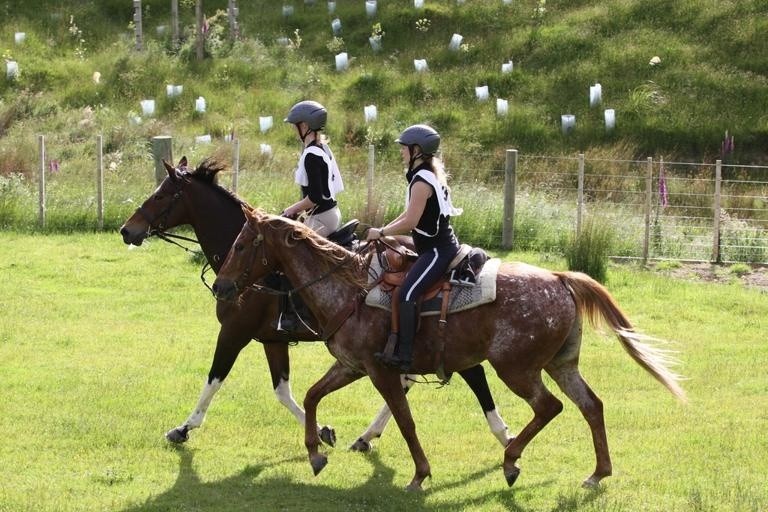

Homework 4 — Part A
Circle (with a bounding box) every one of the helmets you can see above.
[284,100,327,131]
[394,124,441,154]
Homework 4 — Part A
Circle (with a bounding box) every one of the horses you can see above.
[118,156,517,454]
[210,203,697,493]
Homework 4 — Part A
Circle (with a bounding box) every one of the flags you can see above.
[657,157,669,208]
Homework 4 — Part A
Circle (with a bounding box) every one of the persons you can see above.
[361,122,462,373]
[268,99,345,332]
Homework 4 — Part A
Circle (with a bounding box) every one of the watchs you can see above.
[378,227,386,239]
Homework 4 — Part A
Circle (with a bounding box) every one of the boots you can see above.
[373,301,420,373]
